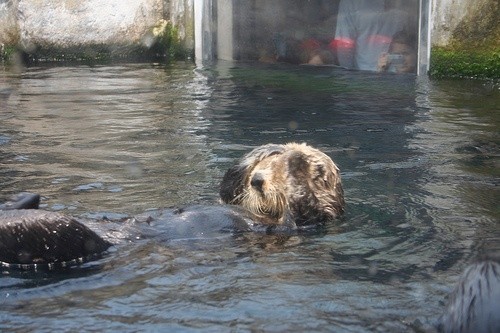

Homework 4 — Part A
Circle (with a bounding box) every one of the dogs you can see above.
[0,143,347,270]
